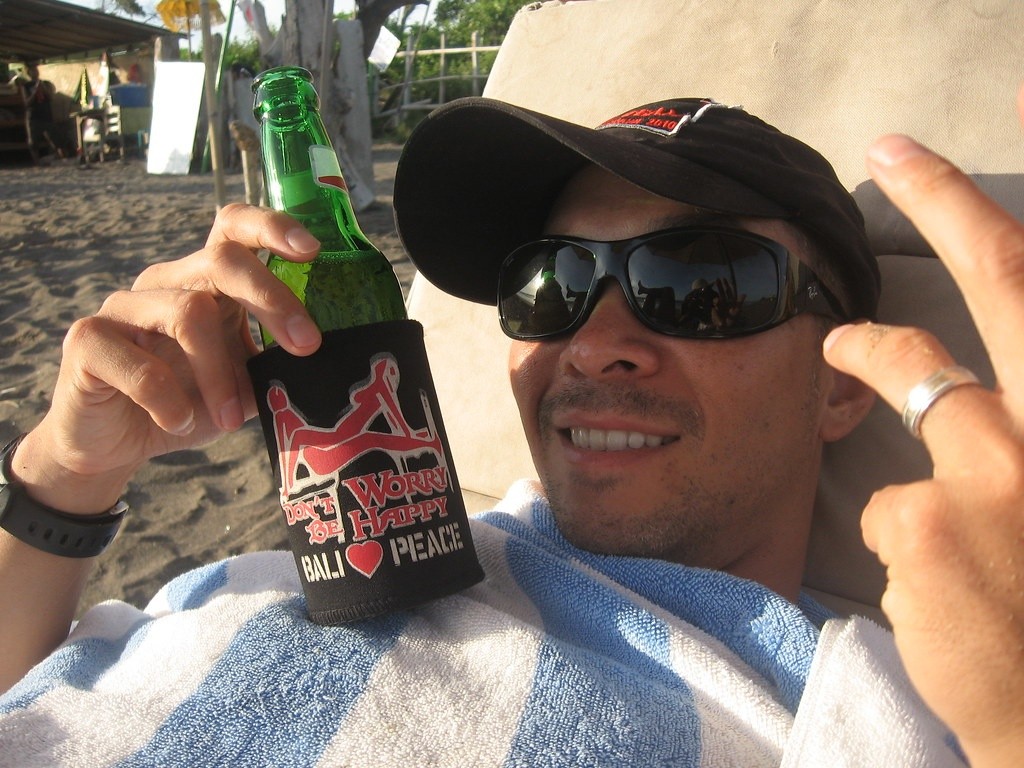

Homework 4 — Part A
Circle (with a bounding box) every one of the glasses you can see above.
[496,225,848,341]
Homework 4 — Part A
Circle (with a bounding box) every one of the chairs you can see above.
[0,85,55,167]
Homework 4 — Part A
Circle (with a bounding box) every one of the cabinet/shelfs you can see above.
[105,105,154,162]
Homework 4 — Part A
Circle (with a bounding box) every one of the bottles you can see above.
[243,65,485,626]
[536,249,563,303]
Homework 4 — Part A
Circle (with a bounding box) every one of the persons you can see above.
[0,98,1024,768]
[22,67,67,166]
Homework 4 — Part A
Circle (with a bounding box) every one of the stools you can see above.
[69,108,106,164]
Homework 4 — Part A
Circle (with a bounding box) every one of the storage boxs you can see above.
[108,81,149,106]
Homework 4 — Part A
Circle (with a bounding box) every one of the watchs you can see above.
[0,432,129,558]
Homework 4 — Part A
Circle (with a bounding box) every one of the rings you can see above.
[902,366,980,439]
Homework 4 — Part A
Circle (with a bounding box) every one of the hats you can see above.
[687,277,716,296]
[392,97,880,324]
